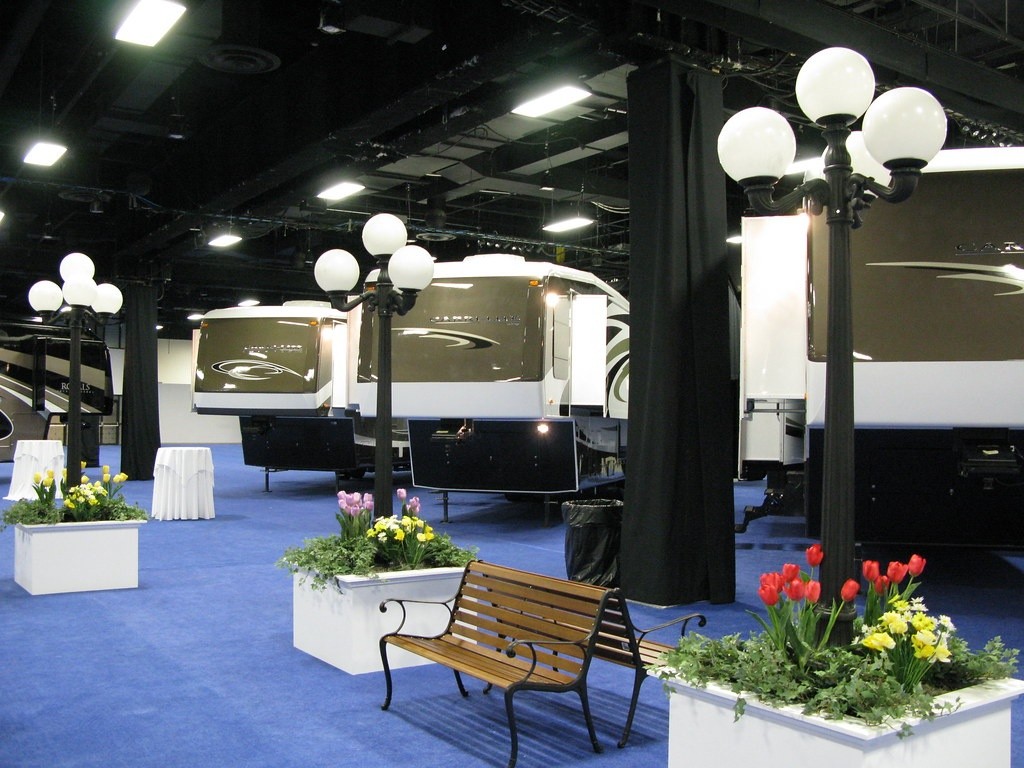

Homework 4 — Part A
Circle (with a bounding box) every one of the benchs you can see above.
[379,557,715,768]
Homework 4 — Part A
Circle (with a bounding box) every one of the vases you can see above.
[648,664,1024,767]
[286,561,478,675]
[12,519,146,596]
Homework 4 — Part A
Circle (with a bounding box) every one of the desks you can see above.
[153,447,216,521]
[7,439,66,500]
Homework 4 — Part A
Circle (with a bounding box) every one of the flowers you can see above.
[5,459,148,525]
[274,489,481,596]
[658,538,1021,743]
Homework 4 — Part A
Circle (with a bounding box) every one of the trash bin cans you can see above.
[81,413,101,468]
[561,497,623,591]
